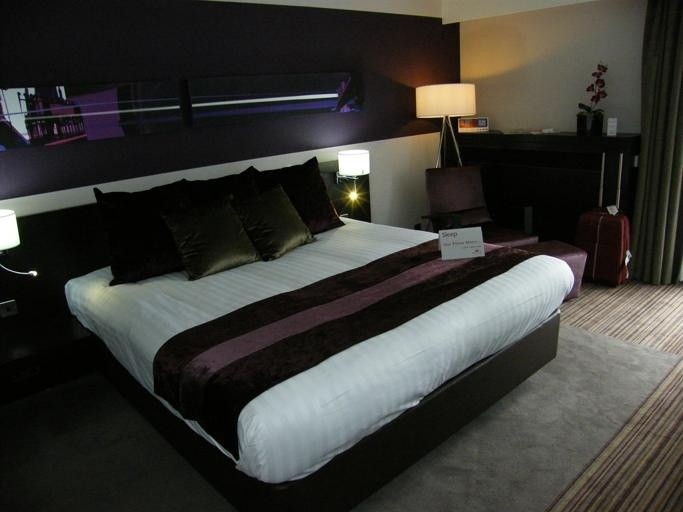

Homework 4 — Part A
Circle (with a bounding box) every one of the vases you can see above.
[577,115,604,135]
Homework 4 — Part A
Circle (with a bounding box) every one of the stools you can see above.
[486,227,588,303]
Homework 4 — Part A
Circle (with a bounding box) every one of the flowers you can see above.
[577,59,608,118]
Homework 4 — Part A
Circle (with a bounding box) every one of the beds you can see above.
[64,214,577,511]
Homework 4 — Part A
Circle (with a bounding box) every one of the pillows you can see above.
[93,157,345,286]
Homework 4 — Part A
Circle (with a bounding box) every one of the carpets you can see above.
[1,321,682,512]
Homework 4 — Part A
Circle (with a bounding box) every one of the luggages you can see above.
[578,149,636,289]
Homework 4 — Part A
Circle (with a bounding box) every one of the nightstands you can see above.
[0,271,68,406]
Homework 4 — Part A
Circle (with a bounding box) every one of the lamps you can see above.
[415,82,476,167]
[335,149,370,184]
[0,209,39,278]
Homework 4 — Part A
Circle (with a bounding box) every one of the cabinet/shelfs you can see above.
[440,131,642,247]
